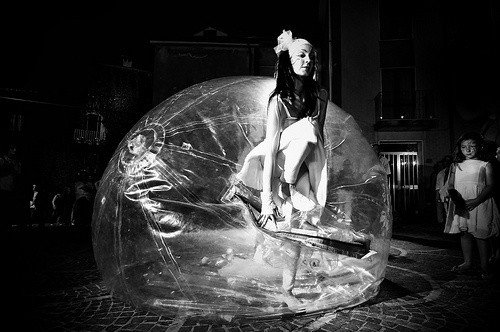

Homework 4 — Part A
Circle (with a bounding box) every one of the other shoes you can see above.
[276,184,317,211]
[280,293,306,313]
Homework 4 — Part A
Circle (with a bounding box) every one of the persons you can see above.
[28,176,101,235]
[433,123,500,279]
[235,29,330,314]
